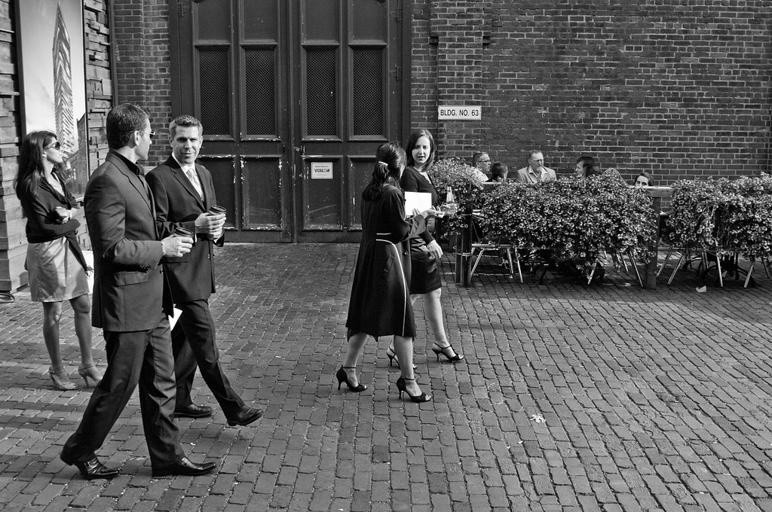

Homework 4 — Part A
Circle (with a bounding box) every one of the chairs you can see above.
[587,249,643,288]
[461,214,523,283]
[657,212,723,287]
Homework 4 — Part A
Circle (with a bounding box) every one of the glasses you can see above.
[140,130,156,138]
[44,142,60,150]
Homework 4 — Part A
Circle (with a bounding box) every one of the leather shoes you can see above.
[152,456,216,476]
[228,405,263,427]
[174,404,212,417]
[61,453,119,479]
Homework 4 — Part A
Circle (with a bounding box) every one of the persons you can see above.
[383,128,464,368]
[335,140,435,403]
[57,100,218,479]
[462,149,656,269]
[144,112,265,428]
[12,130,106,392]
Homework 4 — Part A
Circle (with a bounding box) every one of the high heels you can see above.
[396,377,431,402]
[432,342,464,362]
[387,346,417,369]
[48,368,78,390]
[336,365,367,392]
[78,366,103,388]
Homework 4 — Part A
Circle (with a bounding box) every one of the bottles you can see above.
[446,186,457,215]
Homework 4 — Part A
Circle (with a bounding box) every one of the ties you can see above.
[186,168,205,201]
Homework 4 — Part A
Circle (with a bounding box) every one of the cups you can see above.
[173,226,194,236]
[209,204,226,216]
